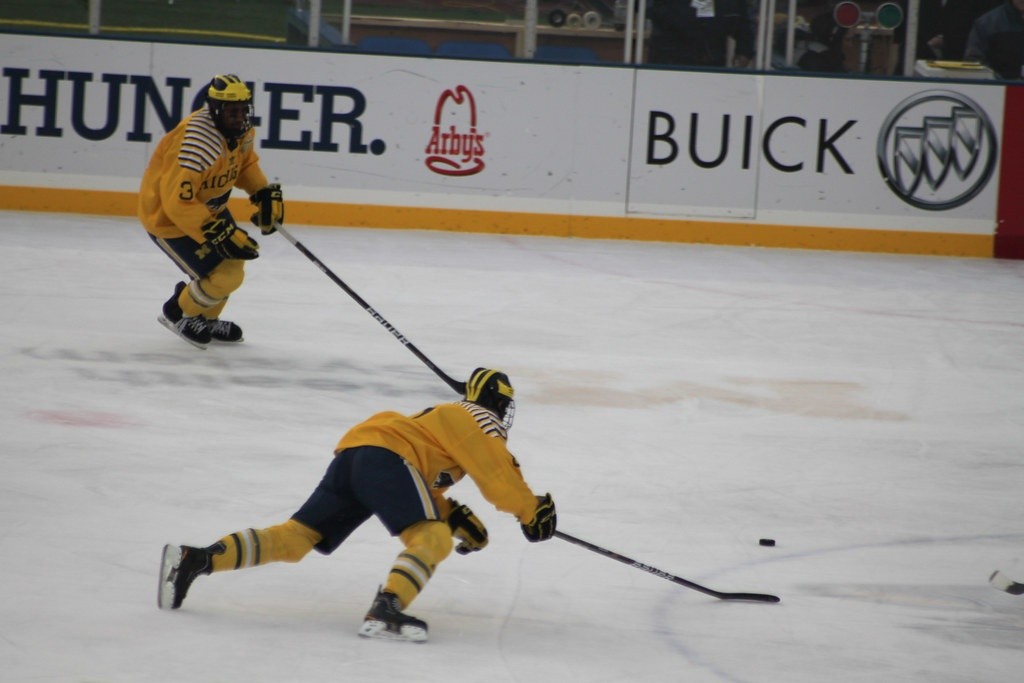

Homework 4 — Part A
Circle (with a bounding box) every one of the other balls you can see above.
[758,539,775,546]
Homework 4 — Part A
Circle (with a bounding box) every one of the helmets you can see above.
[206,73,252,137]
[465,367,516,430]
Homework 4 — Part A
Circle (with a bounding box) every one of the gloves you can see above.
[249,183,285,235]
[200,207,260,259]
[520,492,557,543]
[446,497,489,555]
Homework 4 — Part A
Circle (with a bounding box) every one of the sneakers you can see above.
[156,281,212,351]
[357,584,428,643]
[157,543,213,610]
[207,320,243,342]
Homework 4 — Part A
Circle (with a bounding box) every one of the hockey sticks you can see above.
[274,219,467,396]
[552,524,783,603]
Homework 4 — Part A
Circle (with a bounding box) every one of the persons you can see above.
[890,0,1024,79]
[135,73,285,352]
[633,0,755,69]
[156,367,557,645]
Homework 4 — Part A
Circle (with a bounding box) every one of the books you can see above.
[914,59,994,79]
